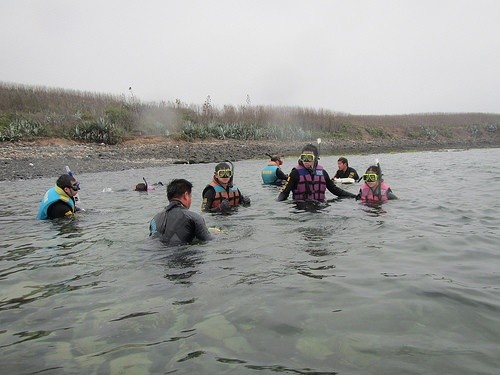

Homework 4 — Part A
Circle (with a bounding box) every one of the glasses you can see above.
[67,182,80,191]
[278,159,283,165]
[299,154,315,161]
[362,174,380,182]
[215,169,232,178]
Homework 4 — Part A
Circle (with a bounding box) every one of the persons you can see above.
[149,179,211,246]
[356,165,398,200]
[261,155,287,185]
[38,175,81,221]
[330,157,359,181]
[276,144,356,201]
[201,162,251,213]
